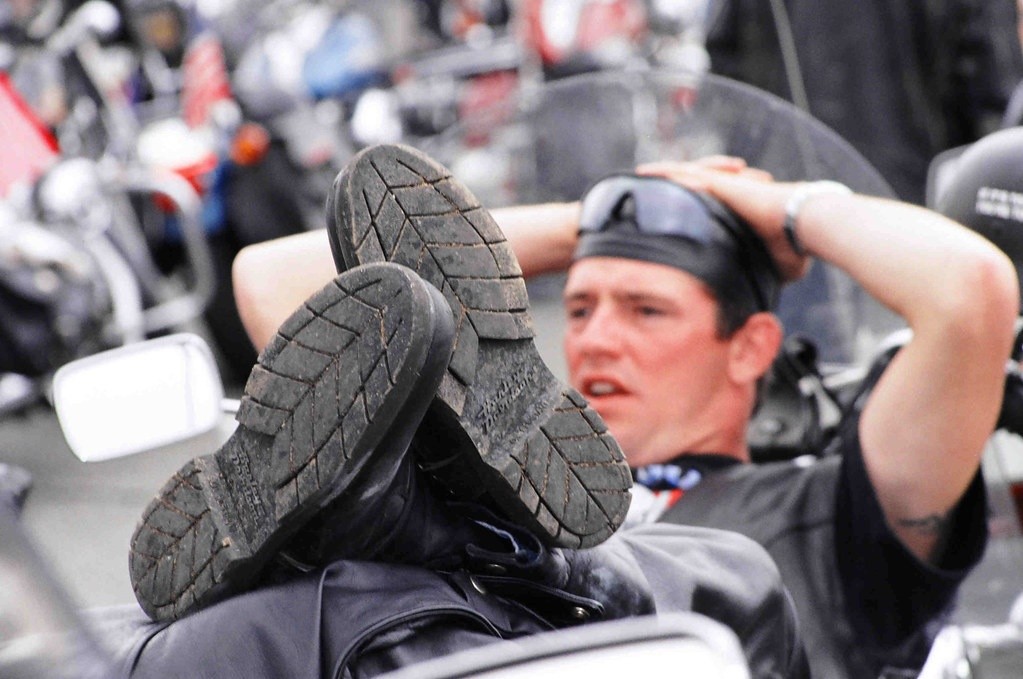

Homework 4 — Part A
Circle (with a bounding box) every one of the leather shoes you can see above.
[326,144,634,550]
[128,261,456,622]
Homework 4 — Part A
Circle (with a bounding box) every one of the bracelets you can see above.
[783,179,852,257]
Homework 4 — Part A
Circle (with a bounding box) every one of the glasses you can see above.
[575,174,737,252]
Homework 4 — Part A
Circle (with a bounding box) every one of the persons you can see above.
[127,142,1018,679]
